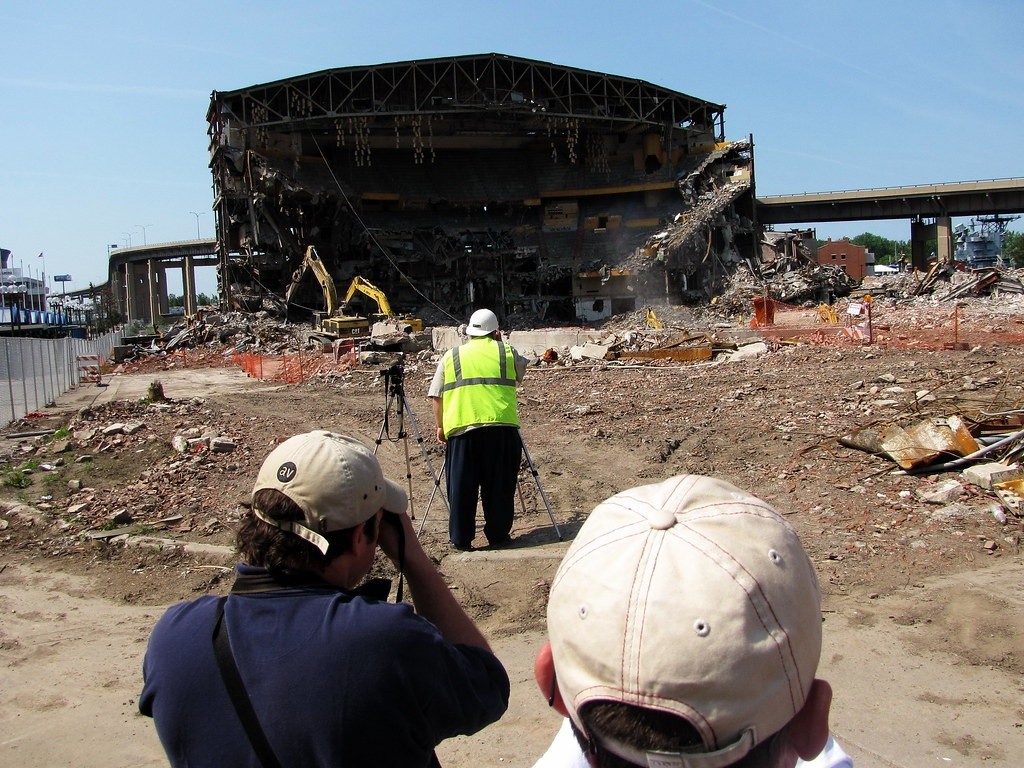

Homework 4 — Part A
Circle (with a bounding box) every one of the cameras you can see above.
[379,364,404,377]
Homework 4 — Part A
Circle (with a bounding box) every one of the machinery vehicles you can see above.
[283,245,373,354]
[345,276,424,333]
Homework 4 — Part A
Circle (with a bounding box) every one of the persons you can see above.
[524,472,855,767]
[136,429,511,768]
[427,307,530,551]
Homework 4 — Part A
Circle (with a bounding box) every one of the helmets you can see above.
[466,308,498,337]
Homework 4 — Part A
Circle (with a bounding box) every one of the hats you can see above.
[251,429,410,556]
[546,473,822,768]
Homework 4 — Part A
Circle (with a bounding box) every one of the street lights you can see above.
[47,297,63,339]
[73,299,86,338]
[85,305,95,340]
[189,210,207,239]
[62,295,77,338]
[135,223,153,247]
[0,274,27,338]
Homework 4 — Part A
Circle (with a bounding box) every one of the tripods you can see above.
[373,377,563,543]
